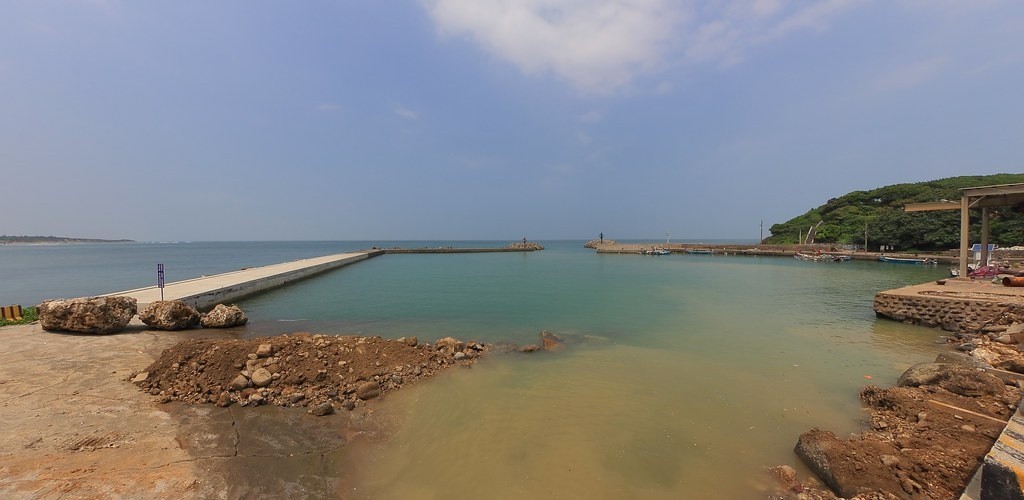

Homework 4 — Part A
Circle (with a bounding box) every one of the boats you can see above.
[638,248,670,255]
[950,263,978,278]
[794,252,851,261]
[876,253,937,264]
[685,248,715,254]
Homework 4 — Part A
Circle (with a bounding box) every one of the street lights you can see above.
[599,232,604,244]
